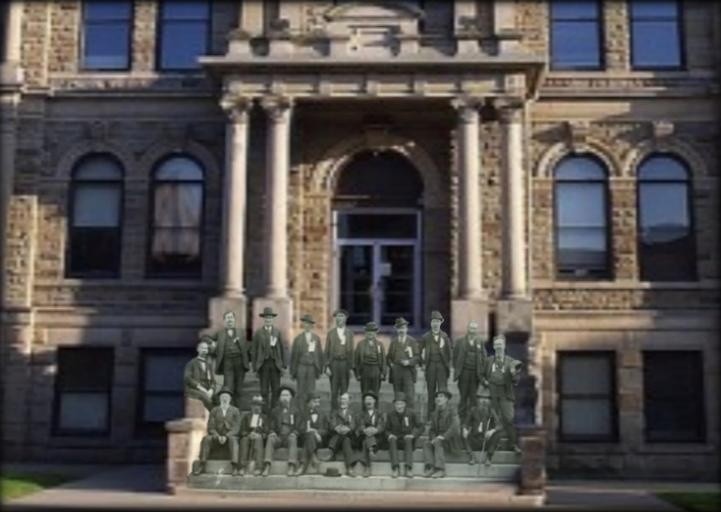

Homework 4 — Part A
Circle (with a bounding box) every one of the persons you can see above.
[184,307,522,479]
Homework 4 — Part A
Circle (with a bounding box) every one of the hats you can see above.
[301,314,314,322]
[259,308,277,318]
[430,311,444,322]
[395,318,407,328]
[217,386,233,396]
[363,322,378,332]
[277,383,295,398]
[393,392,407,401]
[364,391,378,399]
[325,467,340,477]
[250,394,265,404]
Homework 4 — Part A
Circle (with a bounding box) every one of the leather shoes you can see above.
[233,463,270,477]
[392,466,445,478]
[287,463,305,475]
[193,463,204,476]
